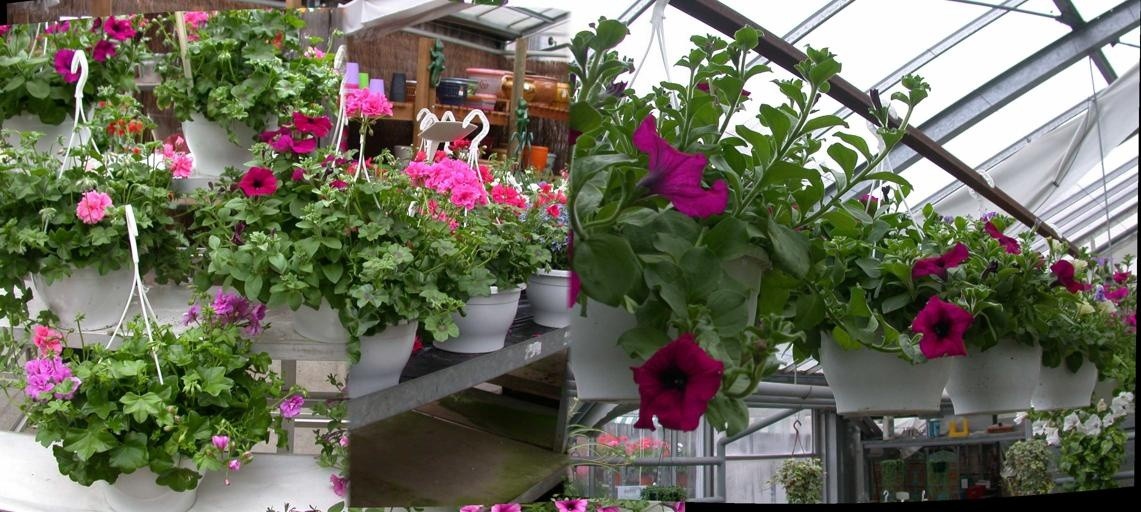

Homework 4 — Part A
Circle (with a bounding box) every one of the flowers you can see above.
[568,17,1137,497]
[0,5,568,497]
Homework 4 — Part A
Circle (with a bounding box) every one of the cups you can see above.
[343,59,416,101]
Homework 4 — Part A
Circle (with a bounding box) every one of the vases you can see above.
[101,456,206,512]
